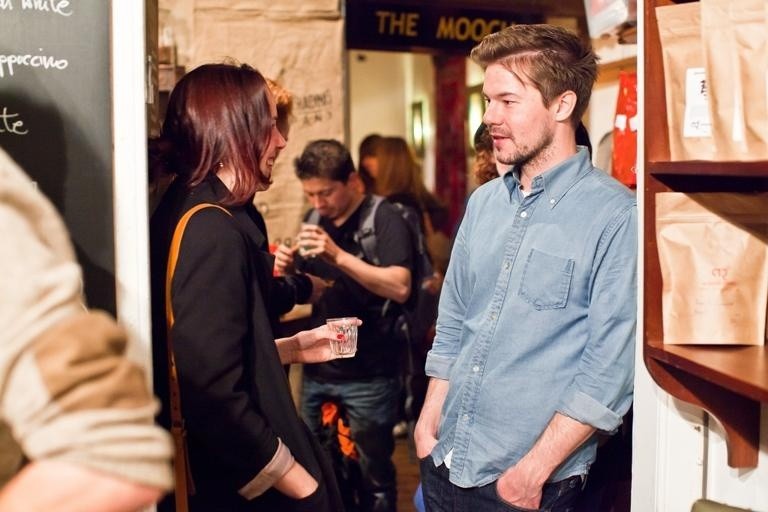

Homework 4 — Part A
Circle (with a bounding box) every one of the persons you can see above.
[407,22,634,510]
[574,119,593,159]
[0,143,177,511]
[150,57,364,511]
[271,273,328,321]
[372,131,445,436]
[273,136,417,512]
[471,125,499,184]
[358,134,384,193]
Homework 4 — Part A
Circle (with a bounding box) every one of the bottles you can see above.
[612,70,640,190]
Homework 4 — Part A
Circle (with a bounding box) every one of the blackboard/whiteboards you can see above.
[0,0,116,319]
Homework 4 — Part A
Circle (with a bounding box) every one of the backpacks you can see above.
[306,192,440,347]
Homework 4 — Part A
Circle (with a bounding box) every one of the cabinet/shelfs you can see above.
[640,1,768,468]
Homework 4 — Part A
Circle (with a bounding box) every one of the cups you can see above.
[325,317,359,356]
